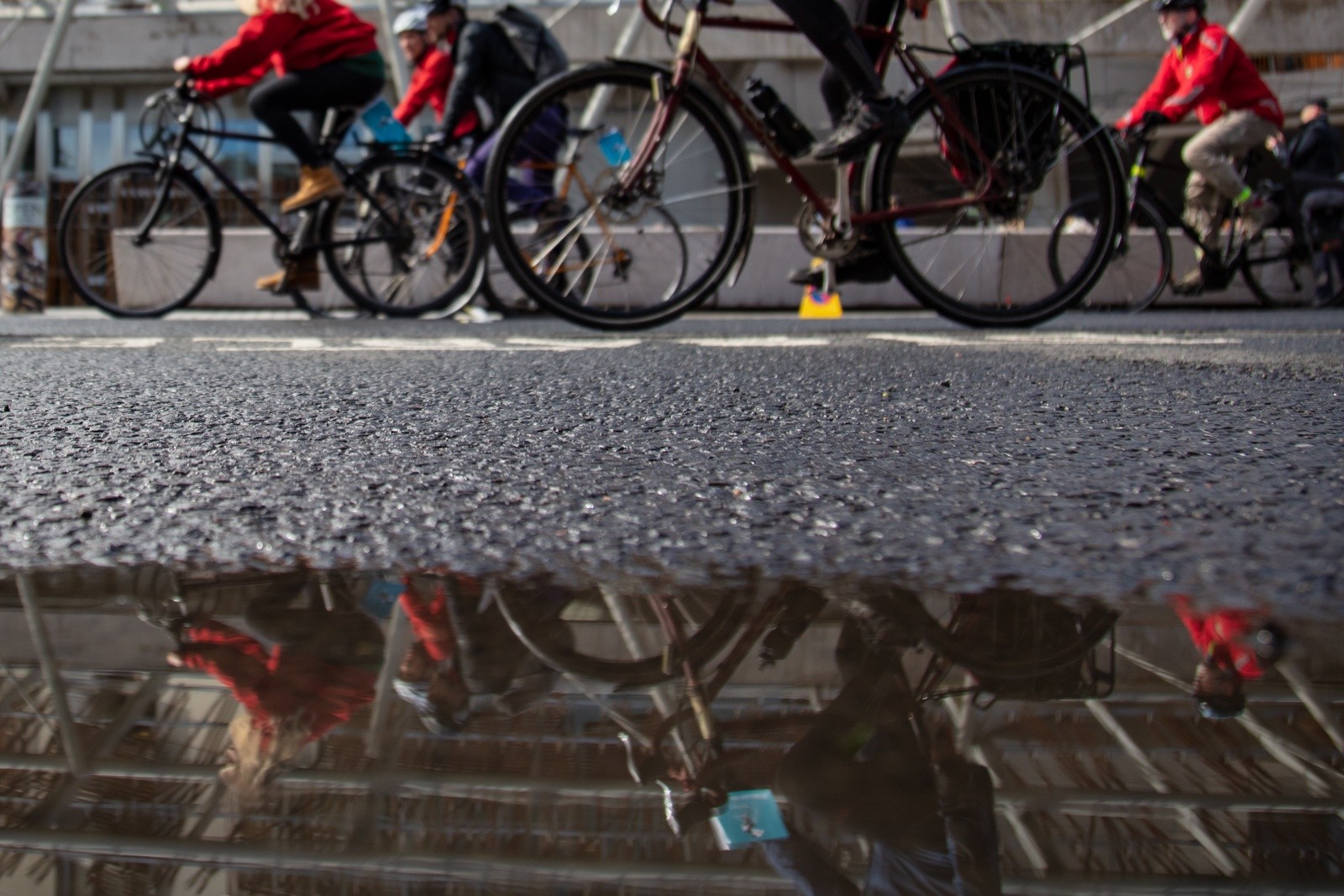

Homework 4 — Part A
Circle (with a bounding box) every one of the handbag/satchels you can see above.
[935,38,1063,194]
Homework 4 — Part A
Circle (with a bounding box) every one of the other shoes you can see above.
[1177,266,1219,292]
[1246,198,1275,240]
[280,165,343,214]
[256,262,320,291]
[533,202,572,243]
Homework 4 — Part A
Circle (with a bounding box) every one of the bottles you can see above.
[744,74,818,156]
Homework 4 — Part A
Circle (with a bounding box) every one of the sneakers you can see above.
[788,246,894,285]
[815,95,894,156]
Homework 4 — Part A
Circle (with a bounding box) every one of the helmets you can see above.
[417,692,479,734]
[392,7,428,37]
[1192,690,1246,719]
[393,677,432,706]
[423,0,468,16]
[1151,1,1207,14]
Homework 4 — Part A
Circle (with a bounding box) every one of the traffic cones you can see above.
[796,251,847,322]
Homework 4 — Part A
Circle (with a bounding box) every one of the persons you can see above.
[1270,100,1331,168]
[174,1,388,289]
[1115,0,1284,286]
[675,600,1002,895]
[392,1,571,242]
[770,0,895,283]
[1173,595,1302,716]
[393,571,577,734]
[166,575,387,792]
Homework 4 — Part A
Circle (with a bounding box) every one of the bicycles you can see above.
[52,67,486,324]
[87,572,1122,839]
[483,0,1133,337]
[279,0,692,327]
[1049,120,1344,313]
